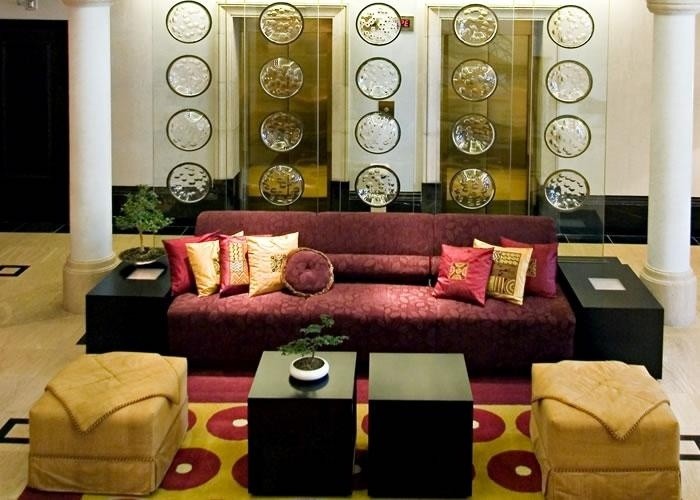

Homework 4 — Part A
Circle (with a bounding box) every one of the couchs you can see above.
[87,209,663,380]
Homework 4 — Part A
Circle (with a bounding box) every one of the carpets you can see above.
[15,367,544,499]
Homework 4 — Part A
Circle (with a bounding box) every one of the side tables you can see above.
[85,256,171,355]
[557,257,664,379]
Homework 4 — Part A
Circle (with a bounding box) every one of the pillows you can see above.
[185,230,244,297]
[280,247,334,297]
[500,236,557,298]
[473,238,534,306]
[216,234,274,296]
[432,244,494,307]
[162,230,220,297]
[246,231,299,297]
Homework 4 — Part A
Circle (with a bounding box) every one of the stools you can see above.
[530,360,682,500]
[28,351,187,496]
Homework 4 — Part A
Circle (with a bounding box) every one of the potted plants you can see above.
[278,313,349,381]
[113,184,175,267]
